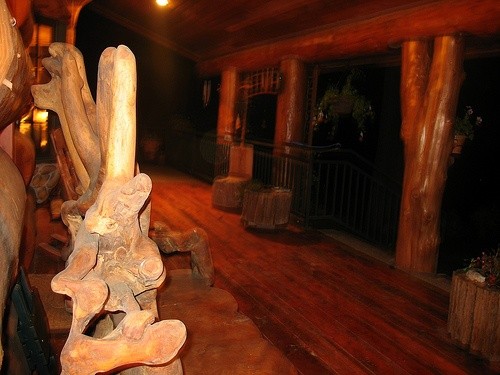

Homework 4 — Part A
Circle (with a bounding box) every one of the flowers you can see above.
[455,104,482,135]
[467,247,500,286]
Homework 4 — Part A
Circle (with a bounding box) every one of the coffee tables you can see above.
[448,267,499,362]
[213,176,245,214]
[243,185,292,231]
[139,135,160,162]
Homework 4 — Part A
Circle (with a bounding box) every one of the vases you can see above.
[451,135,467,154]
